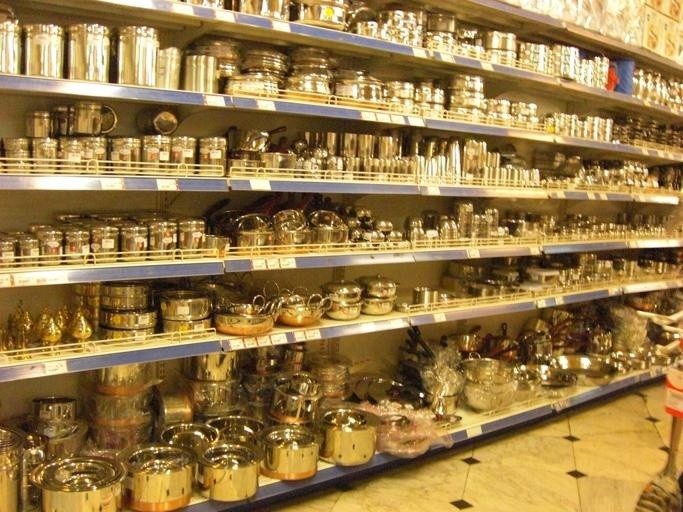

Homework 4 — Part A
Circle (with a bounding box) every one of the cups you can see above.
[1,1,683,268]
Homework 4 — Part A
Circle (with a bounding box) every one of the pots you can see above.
[1,249,683,512]
